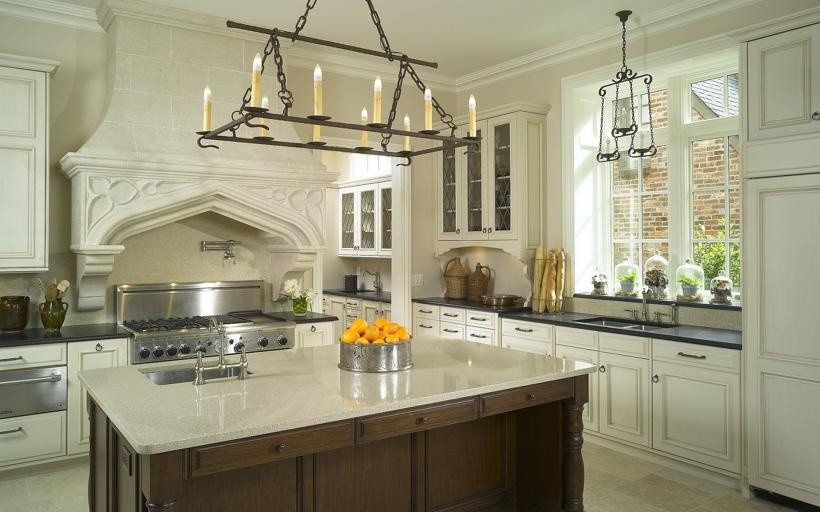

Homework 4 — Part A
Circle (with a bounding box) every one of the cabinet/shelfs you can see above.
[432,111,548,306]
[84,375,587,512]
[741,21,820,139]
[741,141,820,512]
[320,294,391,336]
[0,54,62,273]
[335,180,392,258]
[414,304,498,349]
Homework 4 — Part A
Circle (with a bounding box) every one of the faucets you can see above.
[208,316,226,364]
[641,288,653,321]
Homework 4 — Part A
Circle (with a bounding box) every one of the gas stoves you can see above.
[117,310,296,364]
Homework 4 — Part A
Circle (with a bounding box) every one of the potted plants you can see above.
[279,279,313,316]
[591,257,733,306]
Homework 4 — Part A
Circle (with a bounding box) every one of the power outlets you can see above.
[413,273,424,287]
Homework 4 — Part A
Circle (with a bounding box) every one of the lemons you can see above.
[341,317,410,344]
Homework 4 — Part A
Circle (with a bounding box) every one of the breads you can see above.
[532,245,565,313]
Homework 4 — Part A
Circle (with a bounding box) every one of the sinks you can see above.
[574,317,678,332]
[137,360,254,386]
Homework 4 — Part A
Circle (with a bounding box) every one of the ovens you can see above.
[0,365,68,423]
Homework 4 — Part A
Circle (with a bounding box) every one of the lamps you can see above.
[595,9,657,161]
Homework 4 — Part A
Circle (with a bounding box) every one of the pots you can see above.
[480,294,522,307]
